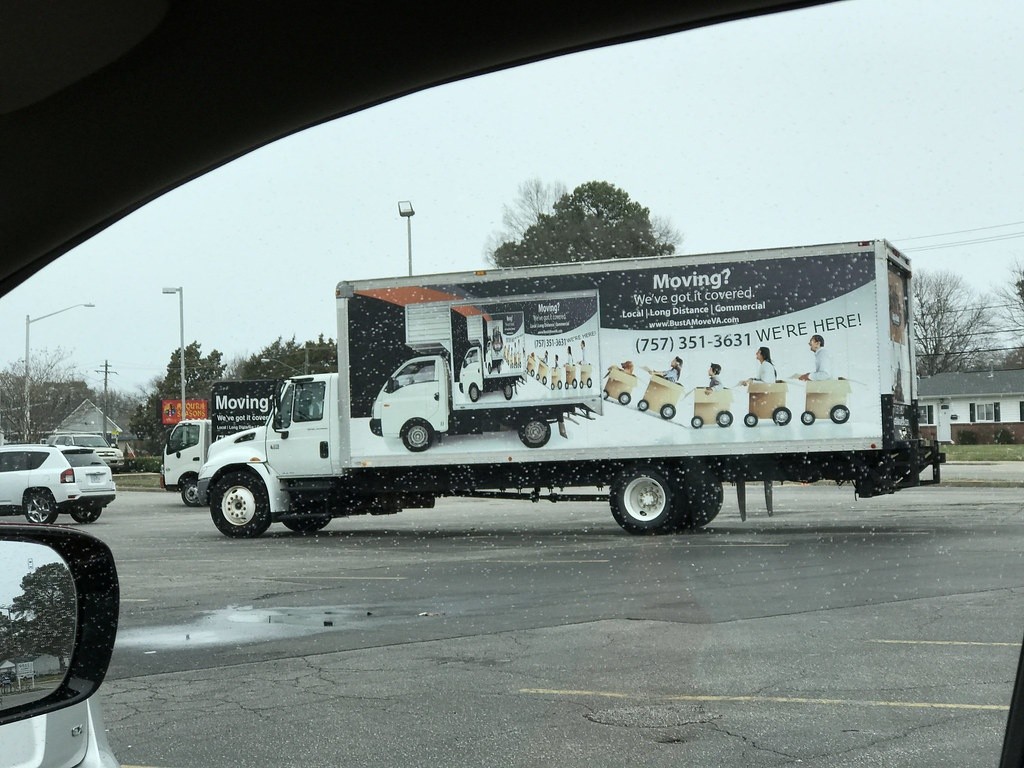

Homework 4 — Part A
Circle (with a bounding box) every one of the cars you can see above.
[46,434,125,474]
[0,444,118,524]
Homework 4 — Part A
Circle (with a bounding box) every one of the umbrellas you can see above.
[1,661,15,670]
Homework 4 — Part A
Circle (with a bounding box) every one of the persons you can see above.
[744,347,777,386]
[705,363,723,395]
[798,334,837,382]
[540,340,588,368]
[508,339,520,355]
[649,356,683,384]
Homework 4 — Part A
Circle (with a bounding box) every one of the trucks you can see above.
[159,378,283,507]
[194,236,918,537]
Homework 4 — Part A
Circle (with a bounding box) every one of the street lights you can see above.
[25,303,95,443]
[162,286,185,421]
[398,200,416,276]
[260,357,308,376]
